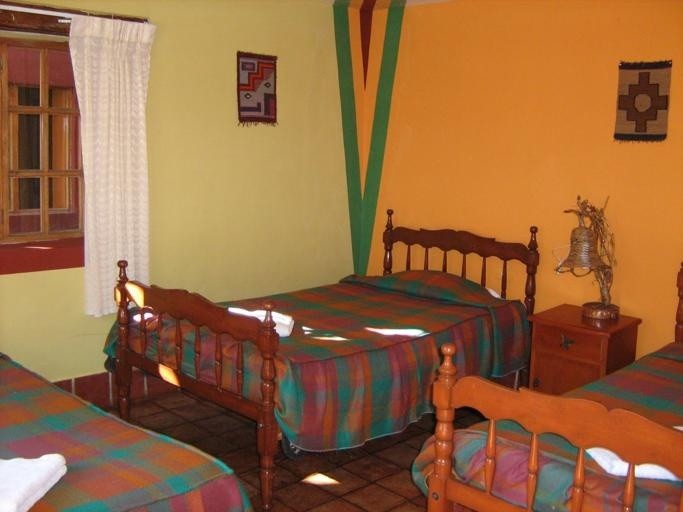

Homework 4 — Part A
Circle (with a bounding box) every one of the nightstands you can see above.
[527,303,643,397]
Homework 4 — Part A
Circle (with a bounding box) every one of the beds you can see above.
[0,353,253,512]
[411,262,683,512]
[114,208,540,512]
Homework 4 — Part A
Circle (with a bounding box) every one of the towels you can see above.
[0,453,67,511]
[586,426,683,482]
[227,306,295,337]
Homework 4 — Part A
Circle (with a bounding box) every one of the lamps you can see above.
[552,194,621,320]
